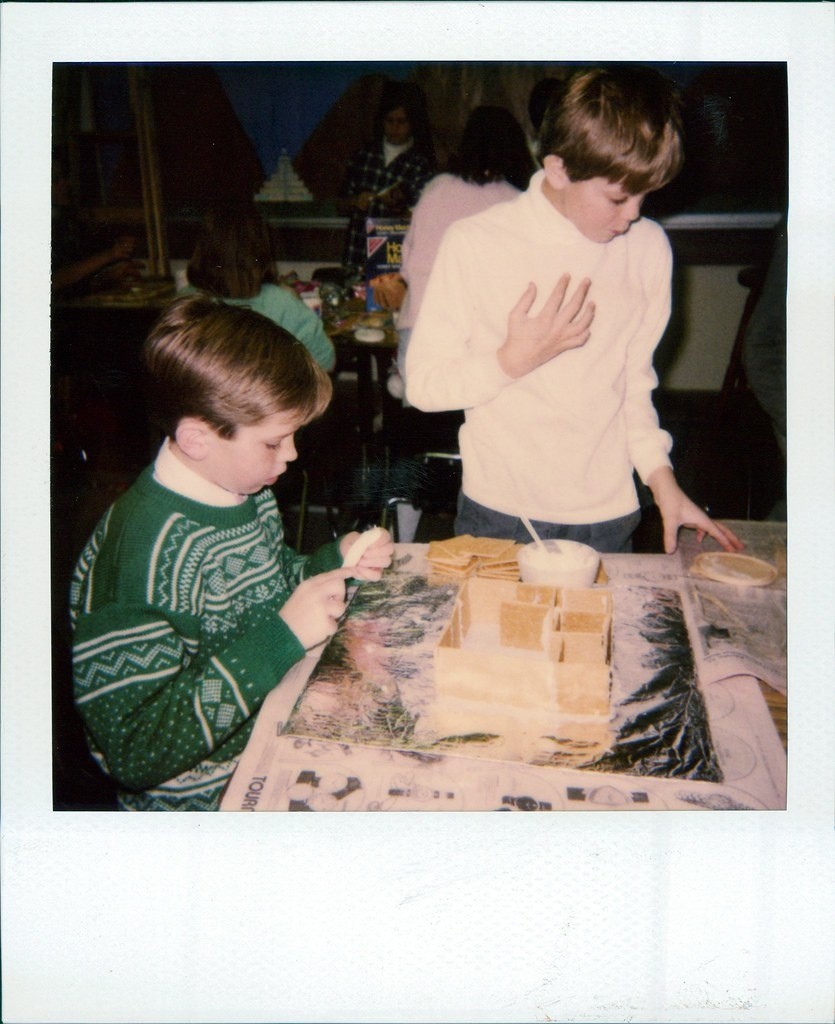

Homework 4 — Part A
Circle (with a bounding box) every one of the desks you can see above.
[217,519,787,811]
[52,277,399,351]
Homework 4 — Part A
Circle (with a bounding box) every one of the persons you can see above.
[344,86,437,282]
[527,77,565,156]
[728,220,786,480]
[354,107,535,544]
[71,297,397,812]
[52,148,165,443]
[157,198,337,503]
[405,70,742,556]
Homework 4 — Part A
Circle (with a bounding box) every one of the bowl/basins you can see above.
[518,539,600,588]
